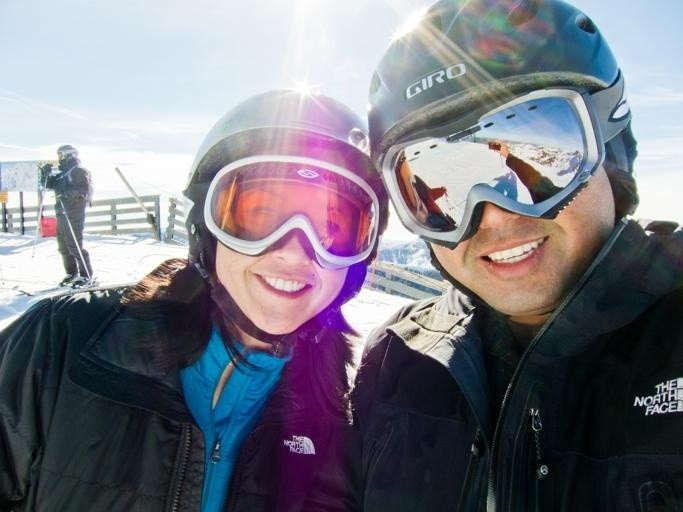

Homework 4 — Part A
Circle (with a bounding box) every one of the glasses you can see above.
[199,154,381,271]
[383,86,606,247]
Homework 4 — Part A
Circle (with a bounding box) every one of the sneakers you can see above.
[63,271,92,288]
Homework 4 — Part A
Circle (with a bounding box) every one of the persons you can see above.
[350,1,683,511]
[0,85,390,511]
[486,138,565,205]
[39,144,93,288]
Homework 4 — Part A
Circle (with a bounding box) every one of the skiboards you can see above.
[18,277,102,299]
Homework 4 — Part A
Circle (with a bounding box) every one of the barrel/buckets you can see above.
[39,216,58,238]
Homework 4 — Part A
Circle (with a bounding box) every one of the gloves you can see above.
[40,164,54,175]
[60,191,77,205]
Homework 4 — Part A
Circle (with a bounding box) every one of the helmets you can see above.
[369,0,631,164]
[184,89,389,230]
[57,146,78,161]
